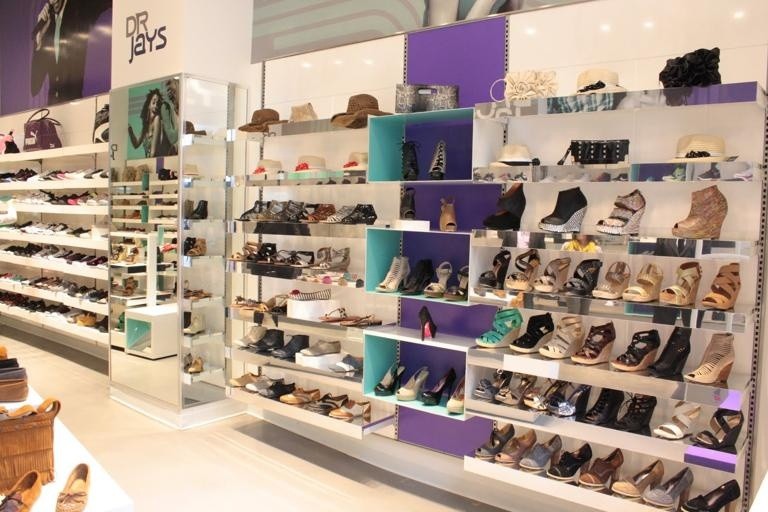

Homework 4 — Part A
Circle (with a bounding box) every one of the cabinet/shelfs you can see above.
[366,106,471,440]
[0,142,111,409]
[111,177,180,361]
[225,120,403,442]
[465,80,765,510]
[185,134,224,385]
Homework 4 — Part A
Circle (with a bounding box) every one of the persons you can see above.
[161,78,179,155]
[30,0,112,106]
[127,89,167,157]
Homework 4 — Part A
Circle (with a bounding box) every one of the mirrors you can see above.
[111,74,181,407]
[183,77,249,404]
[125,316,153,355]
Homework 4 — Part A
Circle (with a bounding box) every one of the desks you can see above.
[0,379,156,512]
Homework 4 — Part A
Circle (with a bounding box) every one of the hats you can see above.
[663,134,739,164]
[293,155,336,174]
[330,94,394,129]
[489,143,542,167]
[572,69,626,95]
[185,121,207,135]
[251,159,283,175]
[290,102,320,122]
[238,109,288,132]
[342,152,368,171]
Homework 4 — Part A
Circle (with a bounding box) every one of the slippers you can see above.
[318,308,360,322]
[190,289,212,300]
[184,288,193,297]
[340,314,382,326]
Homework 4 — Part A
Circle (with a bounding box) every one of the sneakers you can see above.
[11,221,91,237]
[16,190,109,206]
[590,172,611,182]
[539,175,557,184]
[479,173,494,182]
[611,174,629,181]
[507,172,527,182]
[662,168,685,181]
[574,173,589,182]
[558,173,574,183]
[734,170,753,181]
[697,167,721,180]
[492,173,511,181]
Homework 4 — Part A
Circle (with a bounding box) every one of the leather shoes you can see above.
[245,376,284,392]
[309,393,349,410]
[280,387,320,404]
[101,171,110,179]
[271,335,309,359]
[184,353,192,368]
[65,169,88,179]
[300,340,341,356]
[66,253,89,261]
[237,327,267,348]
[258,381,295,399]
[31,244,58,259]
[0,269,69,292]
[328,354,363,373]
[188,356,203,373]
[91,171,106,179]
[57,173,64,178]
[87,256,107,265]
[84,169,103,179]
[67,311,108,334]
[0,358,19,367]
[48,248,74,260]
[329,400,370,419]
[0,367,26,378]
[70,273,108,303]
[44,170,62,179]
[0,470,42,512]
[184,312,191,328]
[2,243,42,257]
[0,172,15,182]
[27,173,45,181]
[0,379,28,402]
[248,329,284,351]
[18,169,36,181]
[56,464,89,512]
[0,292,70,315]
[229,372,262,387]
[184,316,205,334]
[12,168,29,181]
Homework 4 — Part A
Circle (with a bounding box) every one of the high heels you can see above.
[483,183,526,231]
[610,329,661,372]
[397,142,419,180]
[429,141,447,180]
[375,256,411,293]
[611,460,664,498]
[578,448,624,486]
[475,423,515,460]
[681,479,740,512]
[443,265,469,301]
[524,379,575,411]
[374,360,406,396]
[684,333,735,384]
[255,295,287,312]
[614,392,657,432]
[111,245,128,263]
[595,189,646,237]
[659,262,701,306]
[571,321,615,364]
[538,186,587,233]
[519,435,562,470]
[420,306,437,340]
[548,385,592,416]
[547,443,592,480]
[439,196,457,232]
[562,259,603,295]
[495,429,537,462]
[423,261,453,298]
[643,466,694,507]
[509,312,554,354]
[585,388,625,424]
[538,316,584,359]
[504,249,540,290]
[690,409,744,449]
[532,257,572,292]
[654,401,702,440]
[476,308,522,348]
[646,327,692,378]
[592,261,631,299]
[672,185,727,240]
[126,247,139,262]
[473,369,513,399]
[400,259,433,294]
[400,187,416,219]
[700,264,740,310]
[622,262,662,302]
[422,368,456,405]
[396,366,430,401]
[494,373,537,405]
[447,376,464,413]
[479,250,511,290]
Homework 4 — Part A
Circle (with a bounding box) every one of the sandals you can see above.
[242,299,256,310]
[257,250,296,264]
[319,206,355,224]
[235,200,270,221]
[228,241,261,261]
[288,204,319,223]
[184,237,196,255]
[269,200,303,222]
[244,243,276,262]
[185,201,207,219]
[274,251,314,266]
[290,248,332,268]
[185,200,194,217]
[229,296,245,307]
[341,204,377,225]
[299,204,335,223]
[186,239,207,256]
[310,247,350,271]
[270,306,286,314]
[288,288,331,301]
[249,200,288,222]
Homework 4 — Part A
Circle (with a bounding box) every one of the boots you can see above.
[123,278,134,295]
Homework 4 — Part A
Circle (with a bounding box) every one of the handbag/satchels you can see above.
[490,71,558,103]
[92,104,110,144]
[0,398,61,494]
[23,109,62,152]
[557,140,633,165]
[659,47,720,88]
[395,83,459,113]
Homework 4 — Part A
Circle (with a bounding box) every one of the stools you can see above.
[123,303,179,362]
